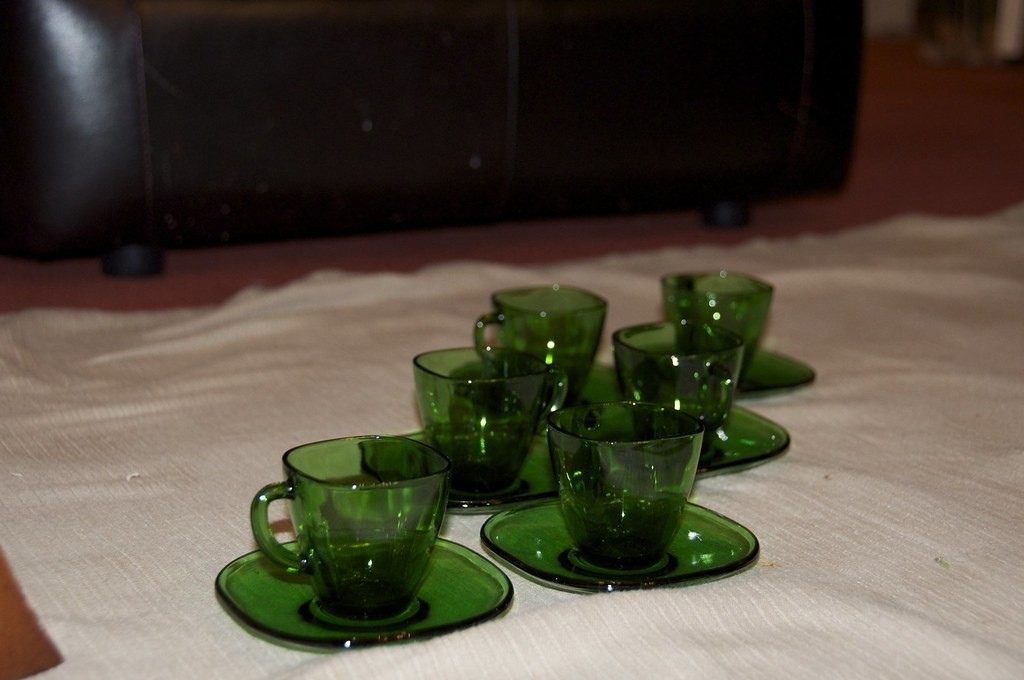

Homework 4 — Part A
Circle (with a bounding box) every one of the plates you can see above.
[581,341,817,482]
[478,494,761,596]
[397,432,560,517]
[215,536,516,650]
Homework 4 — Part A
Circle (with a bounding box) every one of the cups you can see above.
[411,345,567,498]
[612,320,747,463]
[473,285,609,398]
[661,268,775,382]
[250,433,454,620]
[546,401,706,571]
